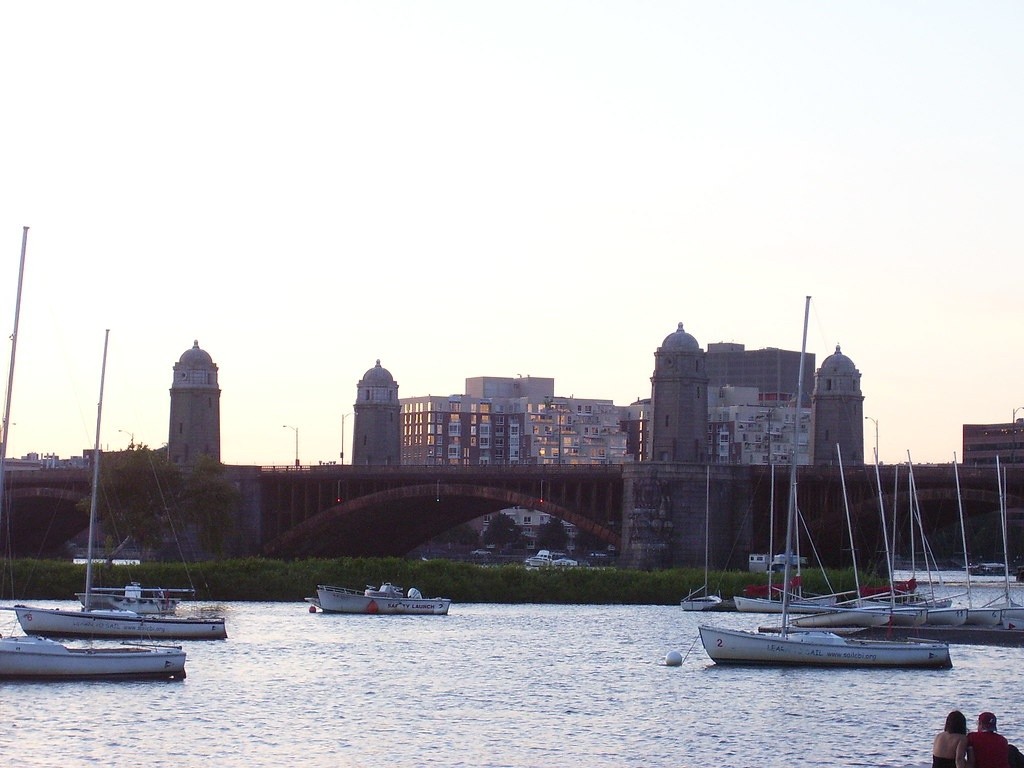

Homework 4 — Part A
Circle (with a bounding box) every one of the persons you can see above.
[932,710,1024,768]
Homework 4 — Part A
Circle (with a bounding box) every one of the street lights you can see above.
[1012,406,1024,470]
[282,425,300,469]
[864,416,879,464]
[339,412,360,465]
[118,429,134,451]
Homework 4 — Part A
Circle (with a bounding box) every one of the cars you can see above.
[472,549,492,553]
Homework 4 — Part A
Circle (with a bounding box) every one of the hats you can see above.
[978,712,998,731]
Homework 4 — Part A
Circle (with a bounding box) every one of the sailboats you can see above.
[696,295,954,669]
[732,443,1024,629]
[12,327,228,640]
[0,225,188,685]
[680,466,724,612]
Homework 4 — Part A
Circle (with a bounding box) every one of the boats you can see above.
[303,582,451,615]
[523,550,578,567]
[72,581,197,615]
